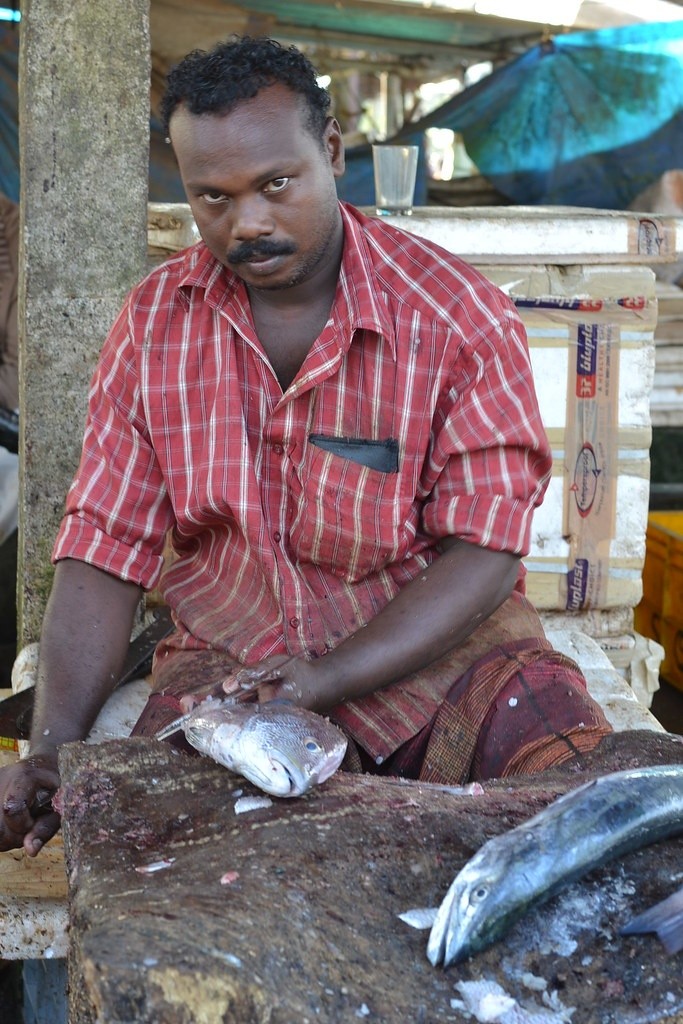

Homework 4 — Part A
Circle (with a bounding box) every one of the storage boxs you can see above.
[469,266,660,605]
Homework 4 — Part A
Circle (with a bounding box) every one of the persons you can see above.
[2,29,617,857]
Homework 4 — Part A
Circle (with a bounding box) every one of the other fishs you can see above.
[427,763,683,973]
[184,694,349,798]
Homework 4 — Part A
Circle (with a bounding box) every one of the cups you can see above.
[372,144,420,217]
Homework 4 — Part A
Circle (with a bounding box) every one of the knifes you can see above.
[32,628,331,827]
